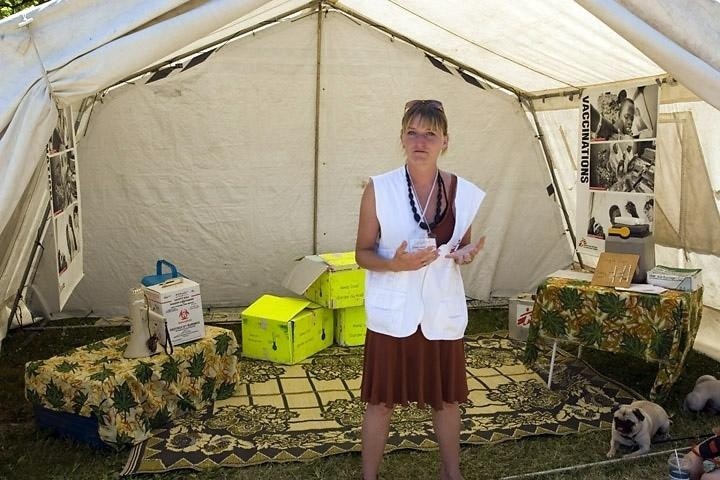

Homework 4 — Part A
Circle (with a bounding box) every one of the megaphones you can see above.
[122,287,169,360]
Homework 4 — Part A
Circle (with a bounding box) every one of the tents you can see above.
[0,0,720,478]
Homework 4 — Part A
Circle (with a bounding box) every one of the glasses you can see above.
[404,100,444,115]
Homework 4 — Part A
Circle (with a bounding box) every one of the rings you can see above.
[423,262,426,266]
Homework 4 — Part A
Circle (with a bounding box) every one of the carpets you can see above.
[118,329,659,478]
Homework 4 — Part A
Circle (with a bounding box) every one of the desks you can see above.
[535,267,704,390]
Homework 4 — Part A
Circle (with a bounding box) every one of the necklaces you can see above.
[405,164,442,229]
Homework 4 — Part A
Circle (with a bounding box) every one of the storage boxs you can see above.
[240,251,367,367]
[141,258,206,346]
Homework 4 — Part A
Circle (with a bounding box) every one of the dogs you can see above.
[605,400,675,460]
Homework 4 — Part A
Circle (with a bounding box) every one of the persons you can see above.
[643,199,654,224]
[609,205,621,226]
[73,206,79,250]
[617,90,635,139]
[622,141,634,172]
[609,142,623,179]
[356,100,486,480]
